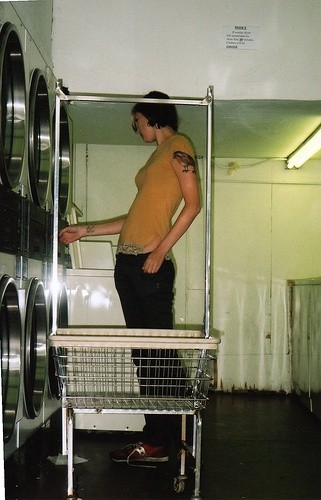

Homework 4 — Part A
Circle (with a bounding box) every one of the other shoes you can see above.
[109,441,169,463]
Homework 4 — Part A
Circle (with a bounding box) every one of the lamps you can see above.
[286,123,321,169]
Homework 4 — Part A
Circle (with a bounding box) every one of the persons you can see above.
[58,90,202,472]
